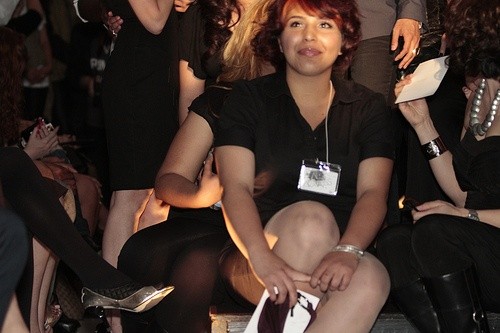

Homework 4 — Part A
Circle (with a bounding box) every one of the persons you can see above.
[0,0,498,331]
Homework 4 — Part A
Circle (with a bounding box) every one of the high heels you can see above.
[79,283,175,329]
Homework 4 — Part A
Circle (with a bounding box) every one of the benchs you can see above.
[207,304,500,333]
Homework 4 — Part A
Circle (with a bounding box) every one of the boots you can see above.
[395,264,492,333]
